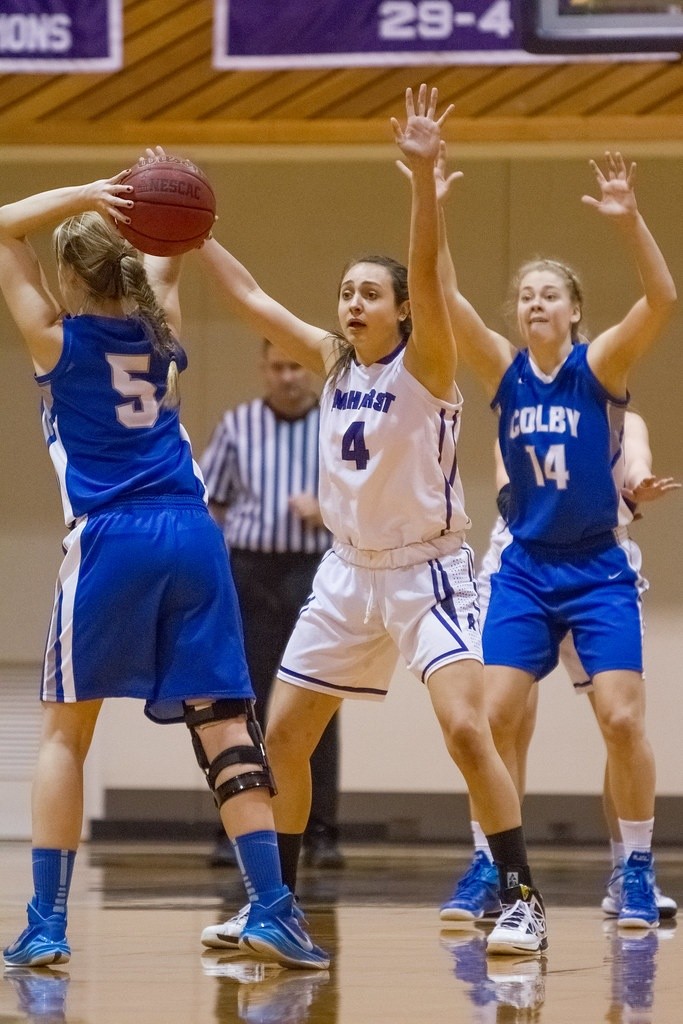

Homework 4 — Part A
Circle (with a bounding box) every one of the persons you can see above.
[196,335,349,872]
[138,83,549,957]
[0,168,332,970]
[396,139,683,931]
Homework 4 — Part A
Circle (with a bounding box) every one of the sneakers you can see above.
[485,867,549,955]
[200,895,299,947]
[487,958,543,1009]
[484,890,504,918]
[617,851,659,928]
[2,901,72,966]
[237,884,330,969]
[619,934,659,1007]
[2,969,70,1018]
[201,949,283,984]
[238,970,332,1023]
[438,849,501,921]
[440,932,489,985]
[601,866,678,919]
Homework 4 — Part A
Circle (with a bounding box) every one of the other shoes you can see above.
[303,824,346,869]
[209,838,238,863]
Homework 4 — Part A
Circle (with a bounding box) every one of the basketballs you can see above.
[110,157,221,259]
